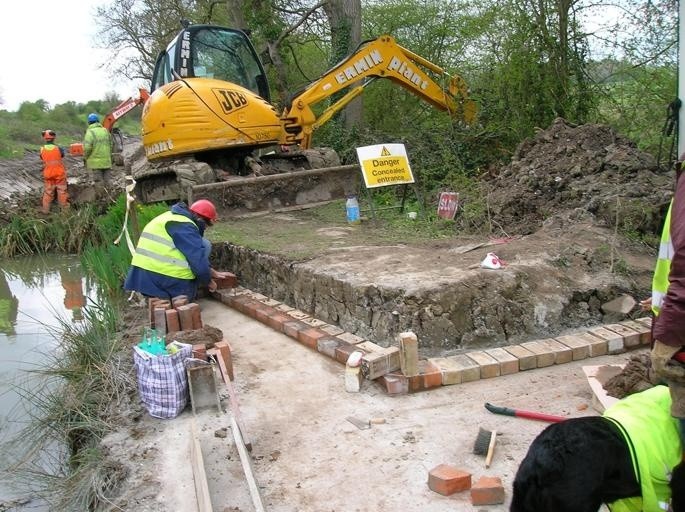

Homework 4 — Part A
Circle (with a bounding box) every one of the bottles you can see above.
[346,196,361,225]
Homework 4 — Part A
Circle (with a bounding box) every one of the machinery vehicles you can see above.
[69,87,152,166]
[130,16,481,206]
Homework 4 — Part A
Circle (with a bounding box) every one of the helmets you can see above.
[88,113,99,123]
[190,199,218,223]
[42,129,56,139]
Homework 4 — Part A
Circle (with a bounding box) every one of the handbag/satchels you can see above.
[133,339,195,419]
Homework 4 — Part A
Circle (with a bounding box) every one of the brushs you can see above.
[473,426,497,469]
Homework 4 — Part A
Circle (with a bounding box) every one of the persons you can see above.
[61,259,88,322]
[81,112,114,197]
[0,267,18,338]
[123,198,238,303]
[39,129,69,213]
[647,154,685,512]
